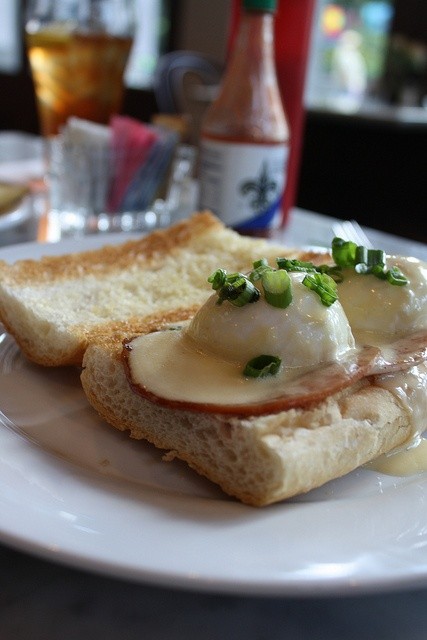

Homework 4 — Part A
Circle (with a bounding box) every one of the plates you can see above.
[1,322,424,601]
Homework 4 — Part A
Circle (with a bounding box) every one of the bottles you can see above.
[198,1,293,238]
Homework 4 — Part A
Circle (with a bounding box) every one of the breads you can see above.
[0,209,426,507]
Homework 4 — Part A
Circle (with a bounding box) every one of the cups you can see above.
[24,18,133,141]
